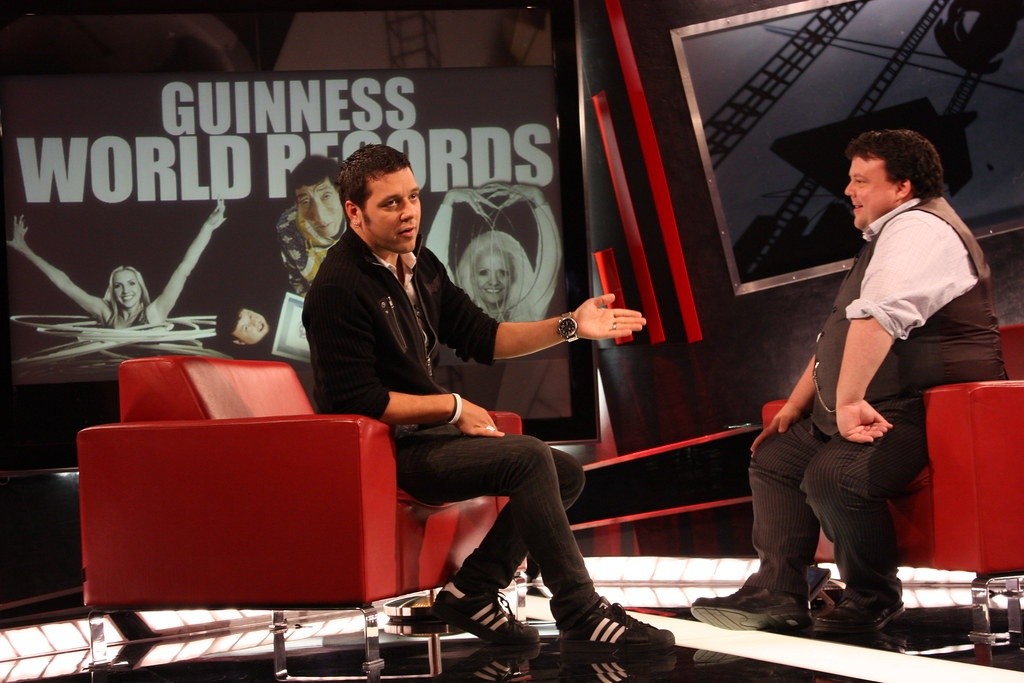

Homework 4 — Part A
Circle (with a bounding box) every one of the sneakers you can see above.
[554,596,675,653]
[430,579,539,645]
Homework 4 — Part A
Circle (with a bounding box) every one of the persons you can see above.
[690,129,1008,629]
[302,145,675,648]
[424,182,563,322]
[7,195,228,330]
[216,155,347,347]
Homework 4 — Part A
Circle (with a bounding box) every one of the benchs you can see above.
[761,323,1024,648]
[78,356,524,683]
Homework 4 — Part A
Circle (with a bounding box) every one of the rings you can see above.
[613,322,617,330]
[486,426,495,431]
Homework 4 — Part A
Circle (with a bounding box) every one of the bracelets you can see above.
[448,393,462,424]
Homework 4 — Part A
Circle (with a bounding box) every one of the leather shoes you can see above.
[690,584,815,639]
[812,597,906,634]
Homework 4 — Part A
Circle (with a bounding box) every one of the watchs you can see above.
[557,312,578,342]
